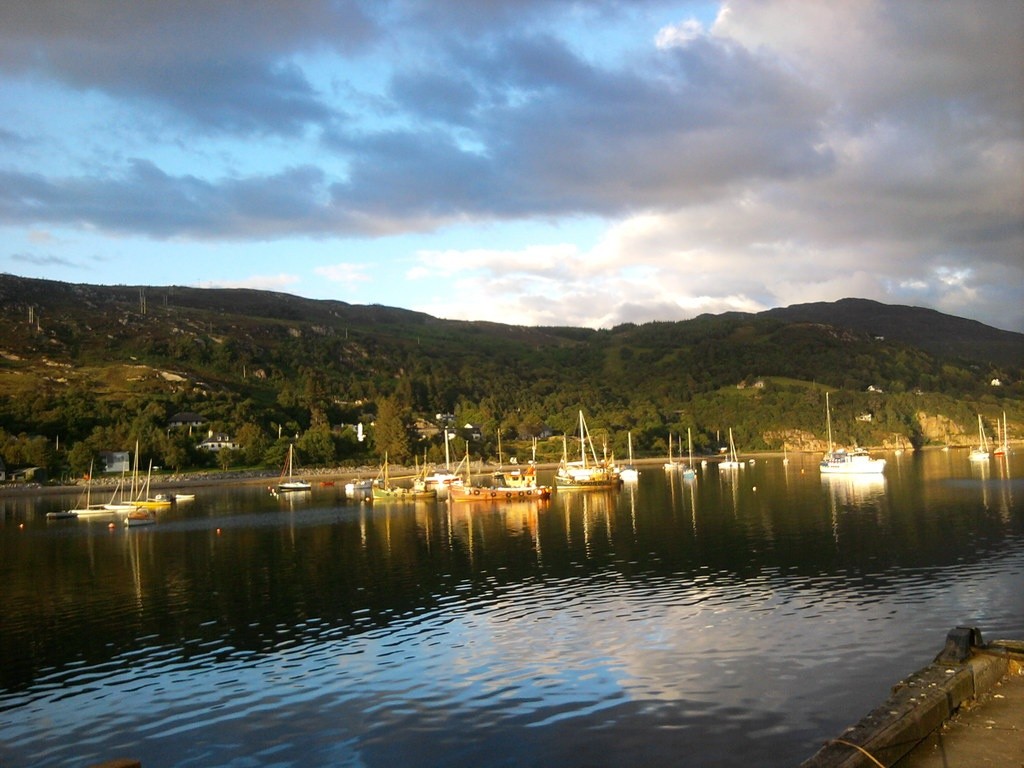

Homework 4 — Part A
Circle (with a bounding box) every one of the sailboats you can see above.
[278,444,312,492]
[663,428,695,479]
[345,426,553,503]
[819,392,888,472]
[718,426,746,470]
[554,409,639,491]
[993,410,1010,456]
[45,438,195,527]
[969,413,991,461]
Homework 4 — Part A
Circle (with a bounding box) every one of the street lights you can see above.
[168,429,172,438]
